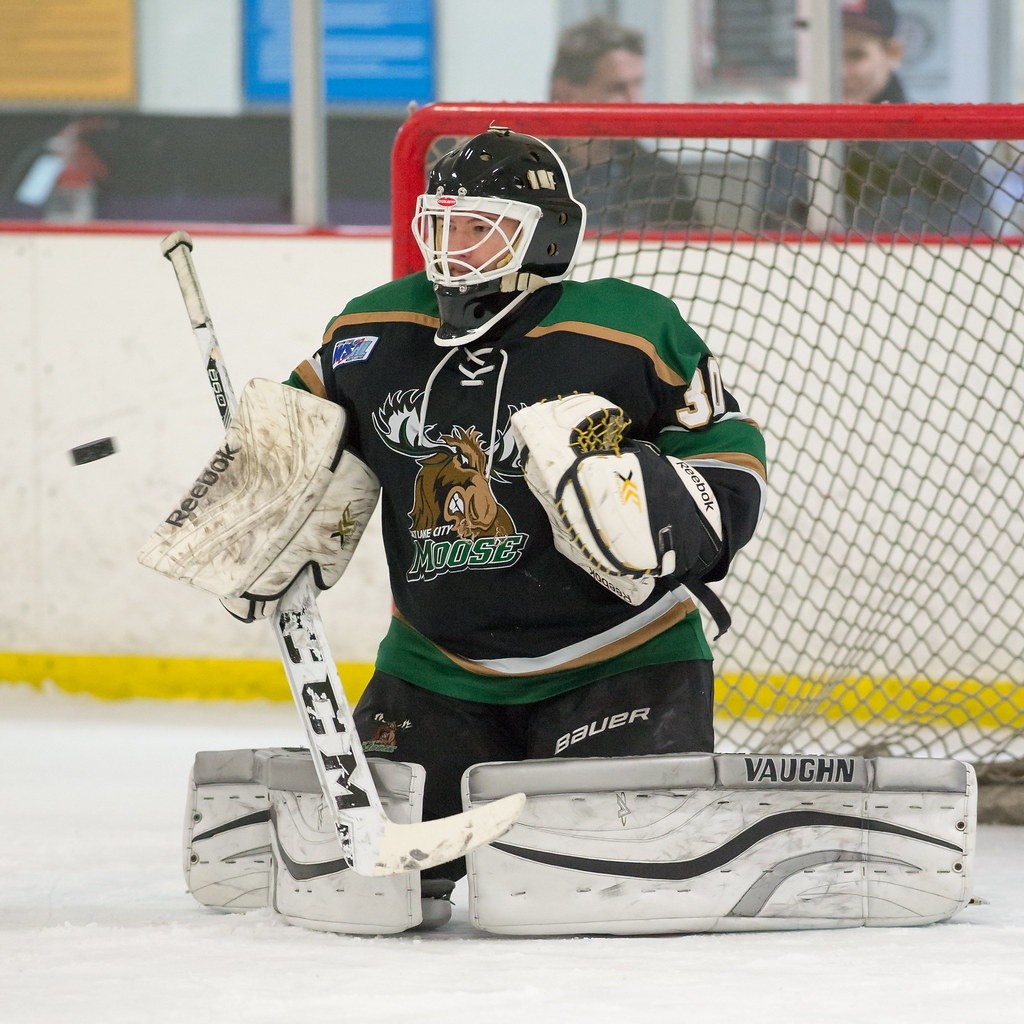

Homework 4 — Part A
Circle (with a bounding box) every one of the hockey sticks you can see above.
[162,231,527,878]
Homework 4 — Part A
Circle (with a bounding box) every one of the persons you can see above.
[217,118,768,936]
[480,16,706,232]
[755,0,997,241]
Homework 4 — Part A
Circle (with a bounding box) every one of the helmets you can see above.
[412,129,587,347]
